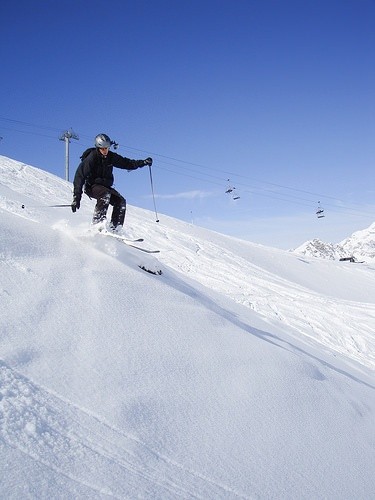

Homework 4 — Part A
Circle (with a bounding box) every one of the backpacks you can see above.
[80,148,96,162]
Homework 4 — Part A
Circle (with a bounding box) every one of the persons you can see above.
[72,134,153,231]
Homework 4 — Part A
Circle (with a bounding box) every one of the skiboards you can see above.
[105,232,160,254]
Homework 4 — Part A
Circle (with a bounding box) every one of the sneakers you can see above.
[92,214,125,237]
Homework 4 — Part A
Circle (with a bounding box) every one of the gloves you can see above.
[143,157,152,166]
[71,194,81,212]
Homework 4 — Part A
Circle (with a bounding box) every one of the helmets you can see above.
[94,134,111,148]
